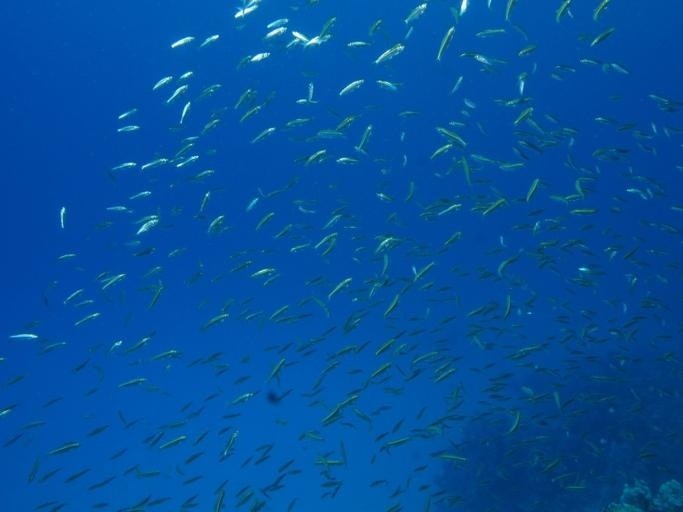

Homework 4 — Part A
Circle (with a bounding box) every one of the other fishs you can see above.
[0,0,682,512]
